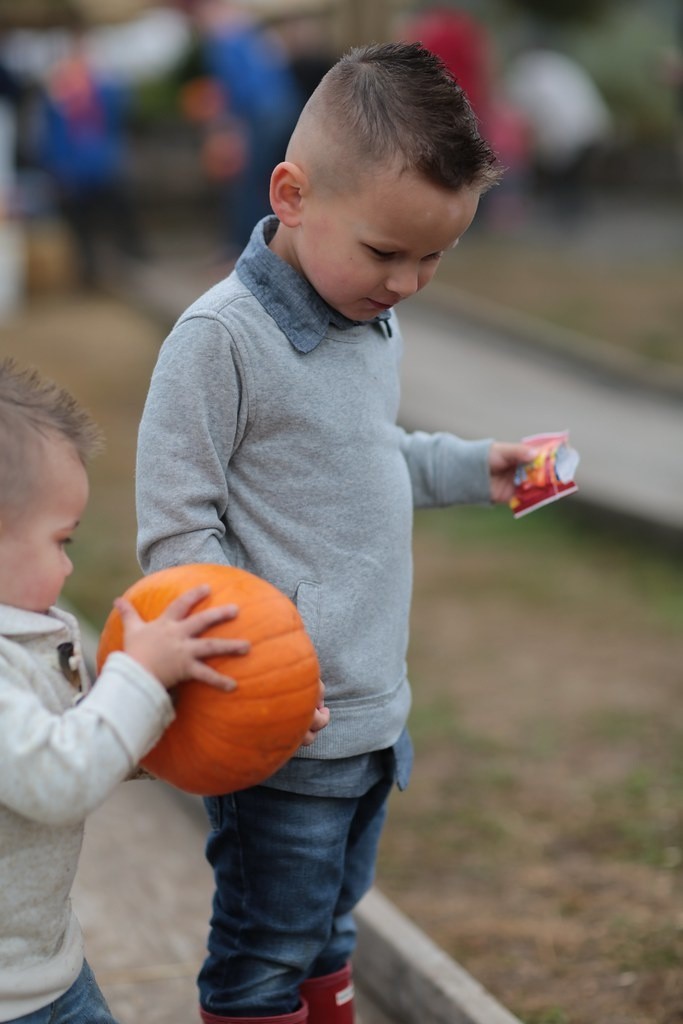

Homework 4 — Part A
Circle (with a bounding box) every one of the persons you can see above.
[1,356,332,1024]
[135,40,536,1024]
[0,0,618,298]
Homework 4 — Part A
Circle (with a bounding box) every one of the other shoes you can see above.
[199,962,354,1024]
